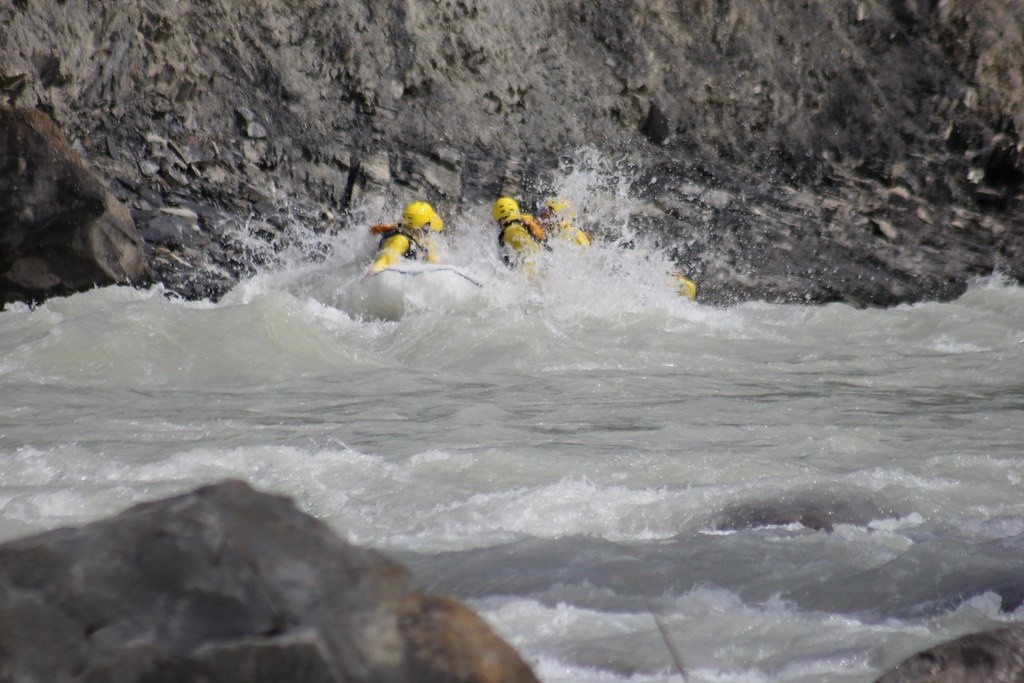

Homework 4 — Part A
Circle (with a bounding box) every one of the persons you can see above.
[492,197,546,283]
[534,199,589,247]
[368,201,443,273]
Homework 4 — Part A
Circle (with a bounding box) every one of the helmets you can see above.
[546,197,567,215]
[402,201,433,231]
[432,211,442,231]
[493,197,524,224]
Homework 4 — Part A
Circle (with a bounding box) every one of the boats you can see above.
[352,263,483,321]
